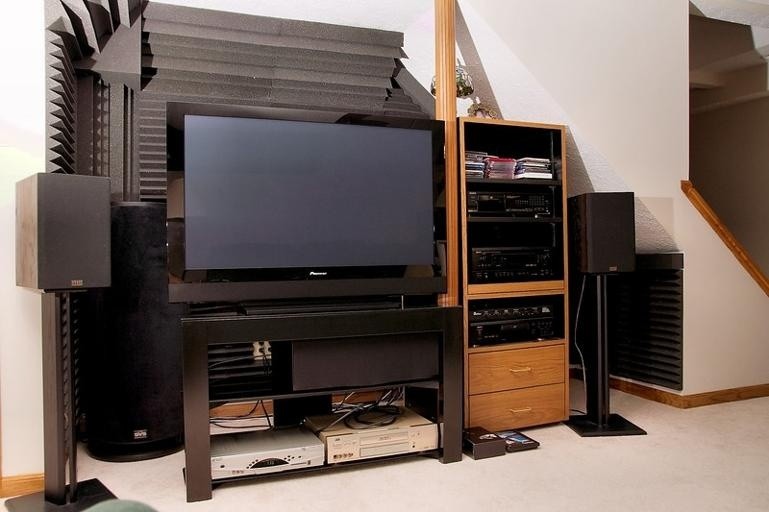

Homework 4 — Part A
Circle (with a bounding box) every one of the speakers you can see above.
[14,172,111,294]
[567,191,636,273]
[74,201,186,463]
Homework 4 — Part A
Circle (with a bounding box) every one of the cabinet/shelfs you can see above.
[459,117,570,433]
[178,306,464,502]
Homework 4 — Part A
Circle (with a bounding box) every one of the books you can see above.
[463,150,553,179]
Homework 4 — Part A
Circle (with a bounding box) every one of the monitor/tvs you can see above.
[164,100,449,315]
